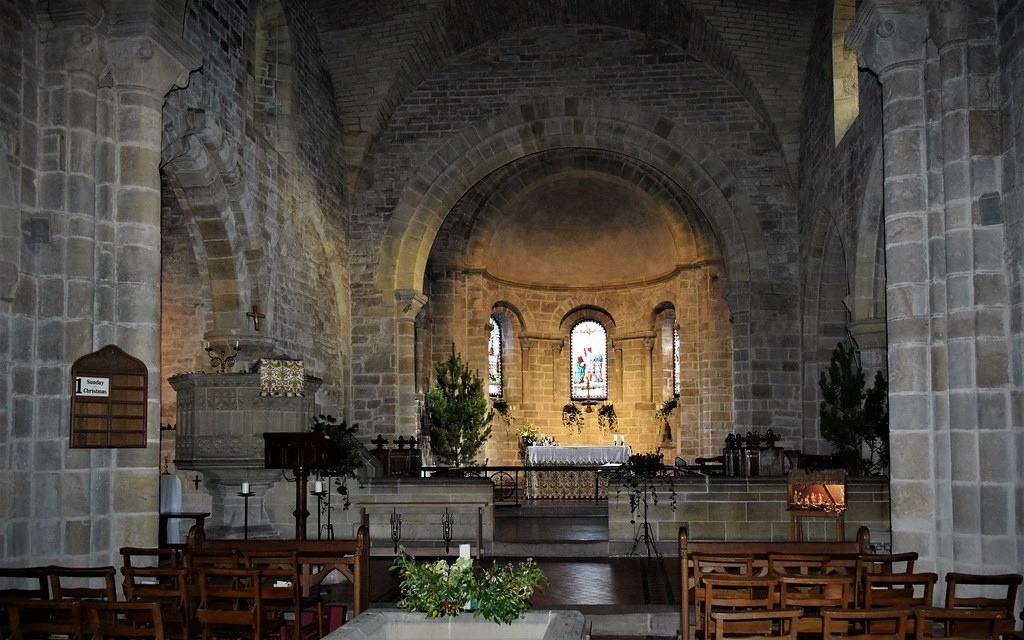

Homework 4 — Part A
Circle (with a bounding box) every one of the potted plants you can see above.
[598,405,619,437]
[562,402,585,435]
[492,398,514,426]
[613,447,677,523]
[388,545,551,626]
[655,397,675,436]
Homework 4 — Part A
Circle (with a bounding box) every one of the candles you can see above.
[614,435,616,440]
[236,340,238,347]
[242,482,250,493]
[316,480,322,492]
[620,435,624,441]
[208,341,210,348]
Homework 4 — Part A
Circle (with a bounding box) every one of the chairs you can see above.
[0,547,322,640]
[691,553,1023,640]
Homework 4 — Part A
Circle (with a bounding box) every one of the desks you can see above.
[354,502,488,560]
[525,446,632,499]
